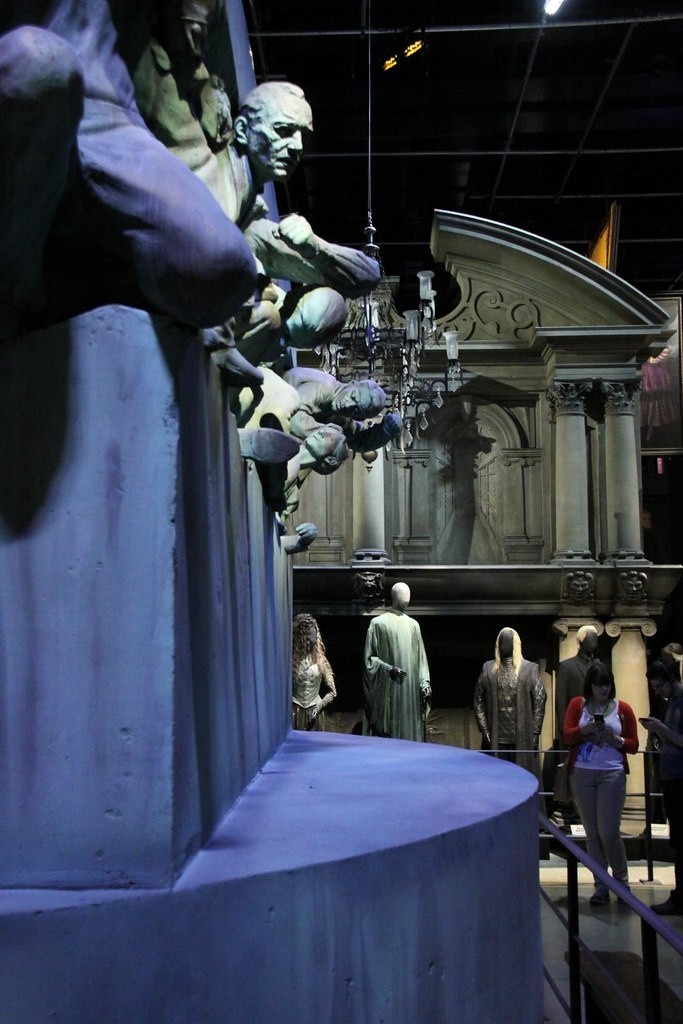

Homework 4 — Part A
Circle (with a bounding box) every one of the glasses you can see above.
[652,680,666,689]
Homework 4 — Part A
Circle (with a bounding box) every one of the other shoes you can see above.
[650,890,683,915]
[618,885,631,904]
[590,889,610,904]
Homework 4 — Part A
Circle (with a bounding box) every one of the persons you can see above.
[639,653,683,915]
[362,581,431,742]
[474,627,547,779]
[543,625,616,834]
[128,0,403,550]
[563,663,639,904]
[291,613,336,732]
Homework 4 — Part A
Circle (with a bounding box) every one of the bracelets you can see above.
[617,737,624,748]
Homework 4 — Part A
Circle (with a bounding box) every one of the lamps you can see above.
[315,2,460,474]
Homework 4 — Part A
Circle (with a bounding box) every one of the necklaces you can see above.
[588,702,608,719]
